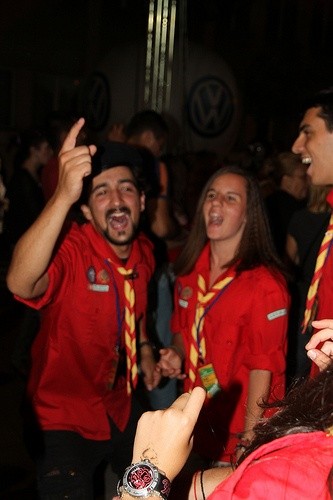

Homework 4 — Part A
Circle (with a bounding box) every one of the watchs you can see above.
[117,460,171,500]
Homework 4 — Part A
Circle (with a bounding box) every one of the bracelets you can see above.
[139,340,155,351]
[194,470,200,500]
[236,436,250,453]
[200,469,206,500]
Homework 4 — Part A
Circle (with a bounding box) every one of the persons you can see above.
[155,163,293,465]
[246,90,332,381]
[117,318,333,500]
[7,117,162,500]
[0,106,186,424]
